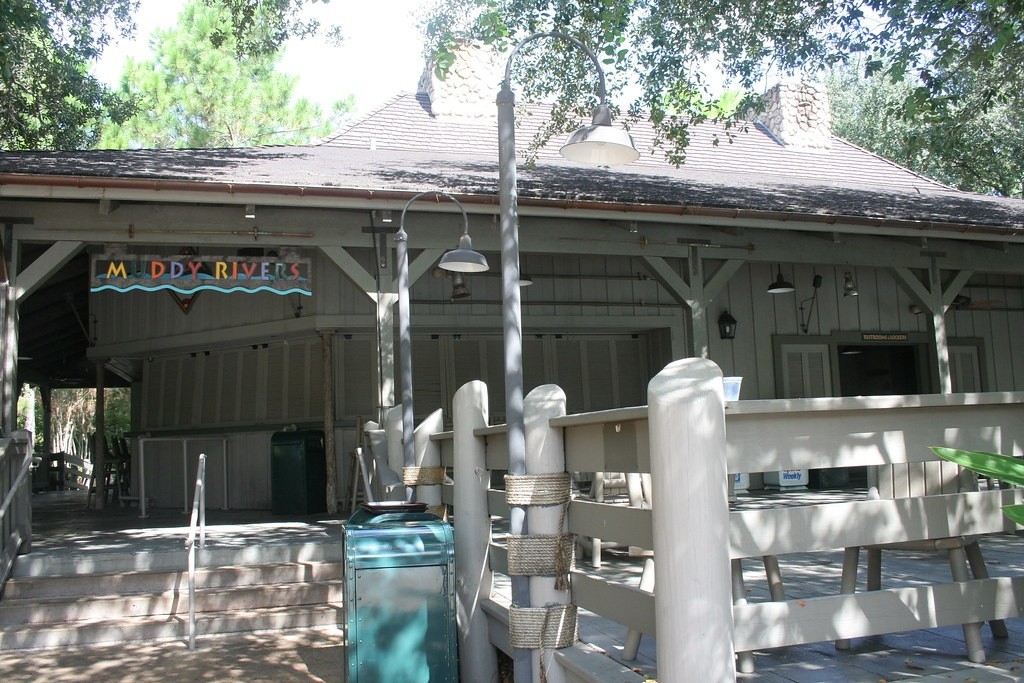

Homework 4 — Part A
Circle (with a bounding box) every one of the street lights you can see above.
[396,190,490,503]
[495,30,641,682]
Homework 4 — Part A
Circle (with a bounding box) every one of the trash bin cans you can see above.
[270,431,327,515]
[348,510,442,524]
[341,520,458,683]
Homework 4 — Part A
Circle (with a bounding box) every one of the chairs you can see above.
[833,461,1007,664]
[574,471,786,673]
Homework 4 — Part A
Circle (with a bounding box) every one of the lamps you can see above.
[765,264,795,293]
[433,267,472,299]
[519,263,533,286]
[717,311,738,339]
[843,272,859,297]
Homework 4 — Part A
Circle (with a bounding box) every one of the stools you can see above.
[86,432,131,507]
